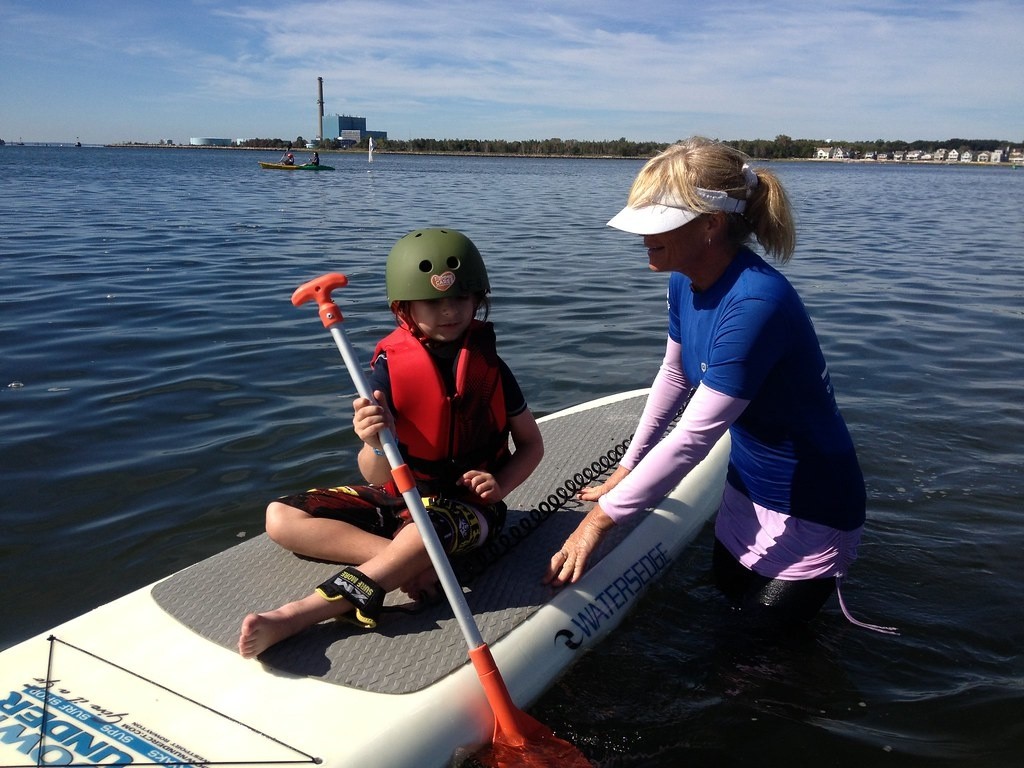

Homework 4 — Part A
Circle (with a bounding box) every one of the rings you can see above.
[560,551,566,559]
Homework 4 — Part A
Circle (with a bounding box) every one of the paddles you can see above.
[289,274,592,768]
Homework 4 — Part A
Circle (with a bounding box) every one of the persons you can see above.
[542,136,867,622]
[304,152,319,166]
[279,153,288,165]
[238,226,545,658]
[283,154,294,165]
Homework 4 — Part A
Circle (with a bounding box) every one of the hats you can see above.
[605,176,748,235]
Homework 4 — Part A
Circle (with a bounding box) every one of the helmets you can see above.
[387,228,490,309]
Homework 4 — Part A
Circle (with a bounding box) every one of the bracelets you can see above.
[373,438,398,456]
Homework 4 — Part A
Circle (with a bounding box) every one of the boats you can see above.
[298,164,336,171]
[258,161,299,170]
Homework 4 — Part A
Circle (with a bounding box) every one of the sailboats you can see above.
[75,136,81,147]
[17,137,24,145]
